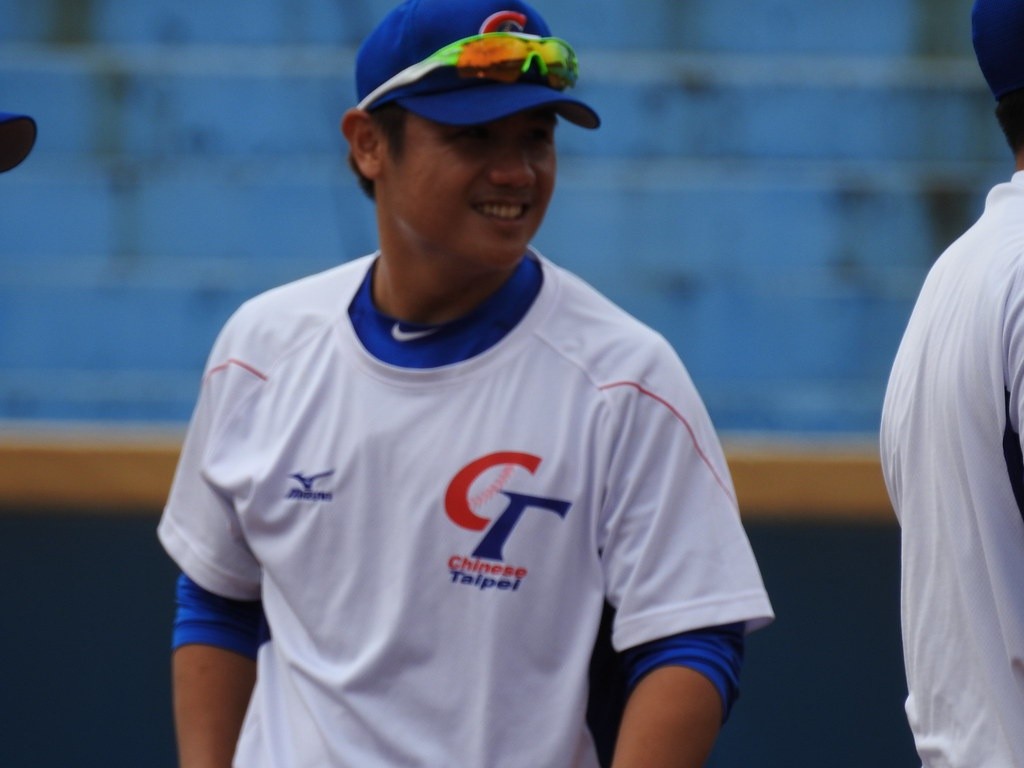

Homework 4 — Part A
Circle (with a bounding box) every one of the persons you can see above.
[880,0,1024,768]
[158,3,773,768]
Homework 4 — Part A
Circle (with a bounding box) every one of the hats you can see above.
[970,0,1024,98]
[356,1,601,131]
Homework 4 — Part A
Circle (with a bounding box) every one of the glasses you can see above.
[357,31,579,114]
